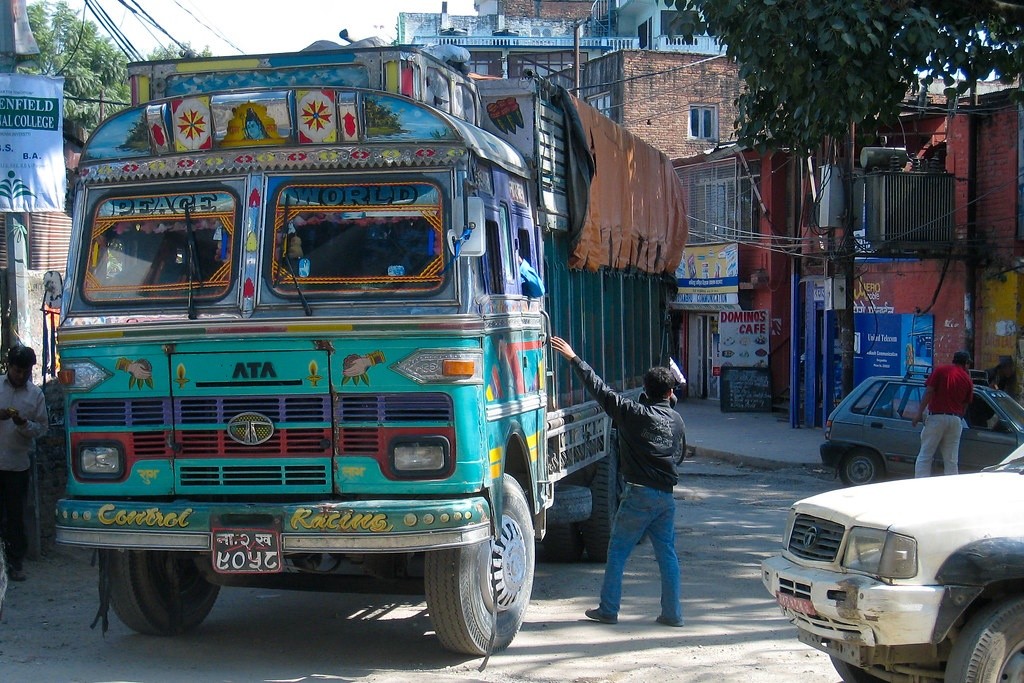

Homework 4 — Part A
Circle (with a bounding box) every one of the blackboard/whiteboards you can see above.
[719,365,773,413]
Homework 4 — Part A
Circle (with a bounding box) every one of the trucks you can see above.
[39,43,687,656]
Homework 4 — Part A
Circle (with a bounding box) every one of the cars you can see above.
[762,442,1024,683]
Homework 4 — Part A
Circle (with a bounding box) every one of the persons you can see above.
[550,335,686,626]
[0,345,48,580]
[518,255,545,298]
[912,351,973,478]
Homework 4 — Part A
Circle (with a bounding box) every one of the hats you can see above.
[954,352,973,364]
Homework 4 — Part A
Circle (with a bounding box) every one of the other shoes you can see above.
[656,615,685,627]
[585,609,619,624]
[8,566,25,580]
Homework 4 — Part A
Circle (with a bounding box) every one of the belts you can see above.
[929,412,962,420]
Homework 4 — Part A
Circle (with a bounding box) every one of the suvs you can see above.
[820,364,1024,488]
